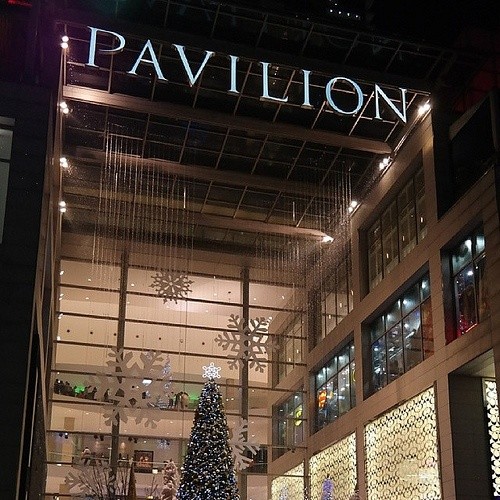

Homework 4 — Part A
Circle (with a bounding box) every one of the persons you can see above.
[244,454,261,473]
[53,373,191,412]
[68,440,183,473]
